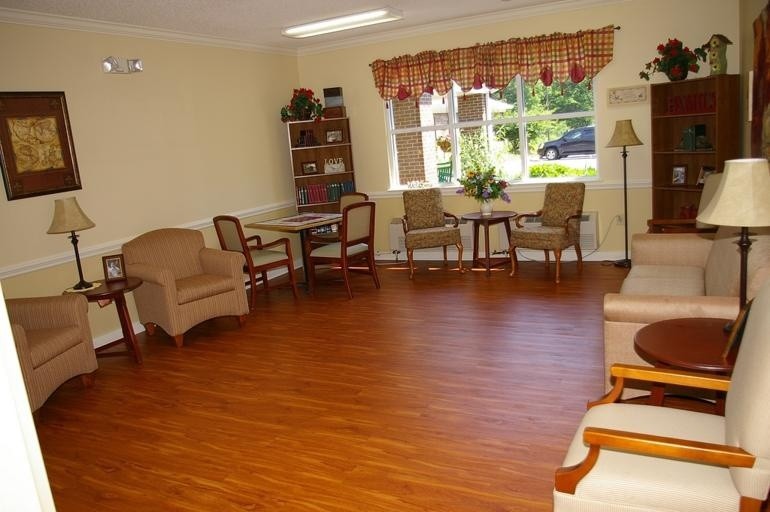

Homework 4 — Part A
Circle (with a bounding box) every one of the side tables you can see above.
[633,317,742,417]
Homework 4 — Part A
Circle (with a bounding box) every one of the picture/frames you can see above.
[300,160,320,176]
[671,164,688,186]
[607,85,649,106]
[0,91,82,202]
[102,253,126,282]
[325,128,345,145]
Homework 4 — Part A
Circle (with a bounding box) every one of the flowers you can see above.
[280,87,323,124]
[456,162,511,205]
[640,38,707,81]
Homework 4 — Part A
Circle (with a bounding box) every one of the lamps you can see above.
[604,119,644,268]
[280,5,404,40]
[44,196,99,292]
[697,158,770,333]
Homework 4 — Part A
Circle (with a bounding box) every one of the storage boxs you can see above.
[323,86,346,118]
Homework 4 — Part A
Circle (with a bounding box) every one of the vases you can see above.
[663,63,689,80]
[298,107,312,120]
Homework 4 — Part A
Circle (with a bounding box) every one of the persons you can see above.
[108,262,120,277]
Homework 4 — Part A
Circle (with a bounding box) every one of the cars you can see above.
[537,126,596,160]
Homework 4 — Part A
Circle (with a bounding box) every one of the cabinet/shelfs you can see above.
[283,118,355,214]
[647,74,739,233]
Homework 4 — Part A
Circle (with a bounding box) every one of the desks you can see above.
[245,210,345,297]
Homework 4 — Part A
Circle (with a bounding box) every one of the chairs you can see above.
[302,200,379,300]
[307,192,372,250]
[554,273,770,511]
[401,183,585,284]
[211,214,303,311]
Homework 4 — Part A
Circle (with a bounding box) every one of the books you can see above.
[295,180,353,205]
[674,123,715,152]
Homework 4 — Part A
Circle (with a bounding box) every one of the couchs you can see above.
[4,292,104,416]
[603,225,770,403]
[119,224,252,350]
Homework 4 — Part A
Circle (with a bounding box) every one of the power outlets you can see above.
[616,215,624,225]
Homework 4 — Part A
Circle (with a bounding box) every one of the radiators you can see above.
[389,215,474,265]
[498,209,599,252]
